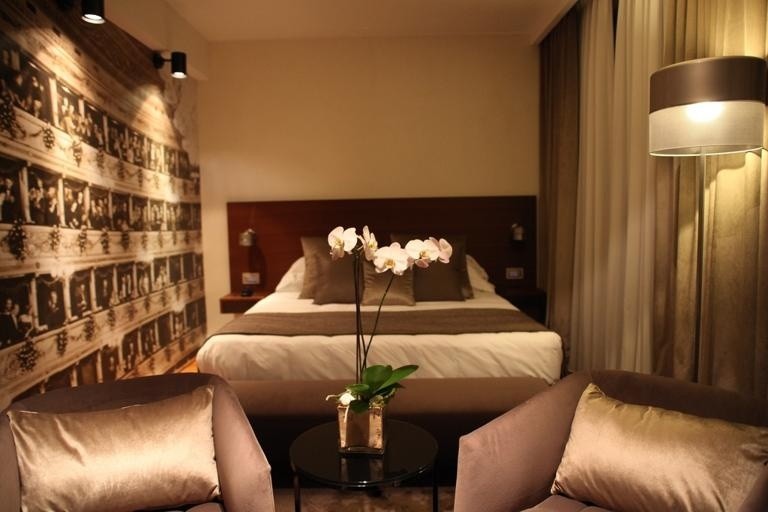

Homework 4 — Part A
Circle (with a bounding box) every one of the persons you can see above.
[3,72,193,179]
[2,254,204,350]
[0,174,203,232]
[104,302,202,380]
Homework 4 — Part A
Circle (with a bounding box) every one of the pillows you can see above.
[277,232,490,302]
[550,382,768,512]
[6,382,223,512]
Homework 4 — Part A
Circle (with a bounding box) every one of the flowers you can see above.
[324,222,453,413]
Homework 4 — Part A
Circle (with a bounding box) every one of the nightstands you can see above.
[220,288,275,312]
[496,285,548,312]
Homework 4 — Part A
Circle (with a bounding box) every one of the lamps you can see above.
[239,228,257,254]
[152,51,187,79]
[648,55,768,384]
[51,0,106,25]
[512,224,523,240]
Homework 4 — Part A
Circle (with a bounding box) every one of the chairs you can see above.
[450,368,768,512]
[0,372,275,512]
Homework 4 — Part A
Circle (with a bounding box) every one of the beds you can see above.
[197,193,571,489]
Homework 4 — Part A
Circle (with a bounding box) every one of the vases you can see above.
[336,403,385,456]
